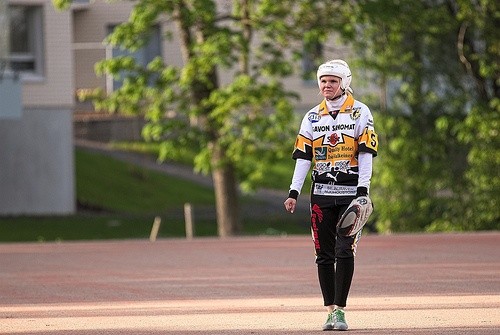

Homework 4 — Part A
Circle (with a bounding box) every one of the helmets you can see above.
[317,60,352,90]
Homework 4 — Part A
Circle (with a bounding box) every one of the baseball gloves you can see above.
[335,195,374,238]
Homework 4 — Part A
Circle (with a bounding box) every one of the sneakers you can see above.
[322,314,333,331]
[333,309,348,331]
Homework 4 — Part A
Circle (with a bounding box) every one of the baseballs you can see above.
[51,0,71,11]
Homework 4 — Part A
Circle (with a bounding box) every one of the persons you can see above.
[284,59,378,330]
